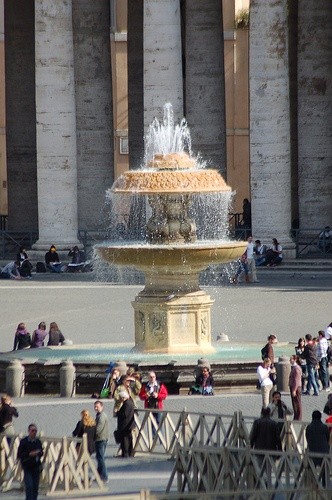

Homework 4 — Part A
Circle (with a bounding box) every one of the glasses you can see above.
[31,430,37,432]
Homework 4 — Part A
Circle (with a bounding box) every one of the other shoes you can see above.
[320,387,330,393]
[121,453,135,458]
[302,391,309,395]
[312,394,318,396]
[104,480,110,484]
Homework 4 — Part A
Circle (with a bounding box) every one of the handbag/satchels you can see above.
[257,380,261,389]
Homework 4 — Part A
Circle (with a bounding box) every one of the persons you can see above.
[241,199,252,240]
[0,396,19,447]
[318,226,332,252]
[112,366,168,445]
[17,424,43,500]
[66,246,86,273]
[249,321,332,488]
[116,391,134,457]
[47,322,65,345]
[45,245,65,273]
[30,321,48,348]
[12,322,32,351]
[1,245,32,280]
[230,236,283,284]
[188,367,214,396]
[72,401,109,483]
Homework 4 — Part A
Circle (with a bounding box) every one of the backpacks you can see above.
[261,344,270,361]
